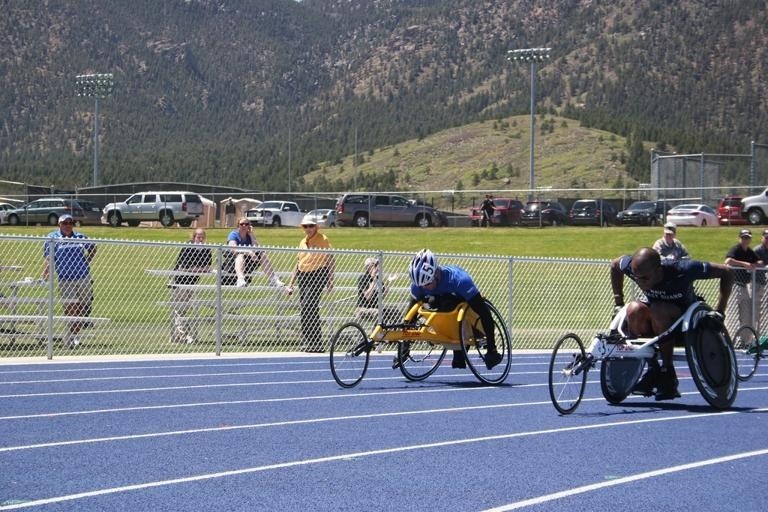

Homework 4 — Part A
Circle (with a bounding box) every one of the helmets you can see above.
[407,247,437,288]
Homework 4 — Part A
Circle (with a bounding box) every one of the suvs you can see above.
[244,193,448,228]
[469,188,768,227]
[0,191,205,228]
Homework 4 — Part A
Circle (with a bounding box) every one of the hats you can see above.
[57,214,74,225]
[739,229,753,239]
[365,258,379,268]
[300,214,318,225]
[663,222,676,234]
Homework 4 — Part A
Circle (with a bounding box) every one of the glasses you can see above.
[302,224,315,229]
[61,221,73,225]
[630,273,647,280]
[240,223,249,226]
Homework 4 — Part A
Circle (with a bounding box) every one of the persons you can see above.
[480,195,496,226]
[169,228,212,344]
[224,197,237,228]
[392,249,501,371]
[221,218,285,288]
[358,256,402,349]
[40,215,98,348]
[287,215,335,352]
[611,222,768,401]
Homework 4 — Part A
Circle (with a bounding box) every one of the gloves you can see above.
[698,309,725,330]
[484,350,503,370]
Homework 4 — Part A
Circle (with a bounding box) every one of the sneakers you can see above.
[632,369,661,394]
[236,276,248,287]
[452,353,467,369]
[656,366,679,397]
[267,276,286,286]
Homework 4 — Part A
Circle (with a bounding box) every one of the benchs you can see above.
[146,269,412,342]
[0,265,111,349]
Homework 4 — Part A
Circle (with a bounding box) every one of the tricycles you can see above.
[547,300,737,415]
[732,324,768,383]
[327,295,513,389]
